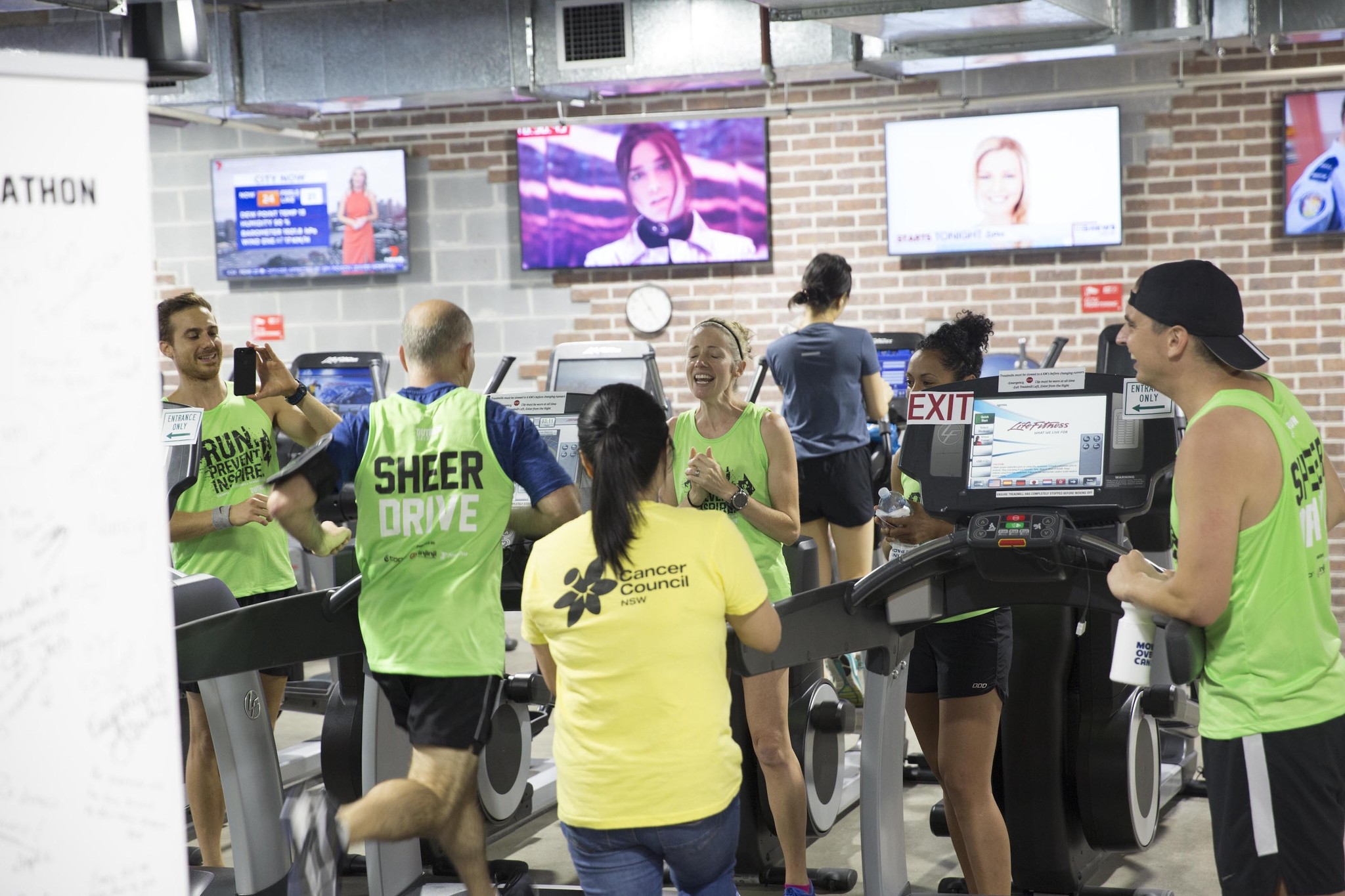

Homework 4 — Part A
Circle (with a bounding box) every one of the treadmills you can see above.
[160,323,1209,896]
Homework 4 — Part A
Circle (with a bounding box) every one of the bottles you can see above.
[878,487,912,516]
[1109,601,1156,687]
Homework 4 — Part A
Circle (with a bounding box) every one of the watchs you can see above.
[725,485,749,513]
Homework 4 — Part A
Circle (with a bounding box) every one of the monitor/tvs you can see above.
[295,364,377,413]
[883,104,1123,255]
[516,116,772,272]
[855,344,917,443]
[551,347,649,397]
[962,395,1110,488]
[1280,89,1345,237]
[210,147,411,281]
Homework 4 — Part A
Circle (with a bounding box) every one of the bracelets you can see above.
[687,491,704,507]
[212,504,234,530]
[284,379,307,405]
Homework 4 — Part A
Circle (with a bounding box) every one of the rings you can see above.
[695,470,700,477]
[267,358,271,360]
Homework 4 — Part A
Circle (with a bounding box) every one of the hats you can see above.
[1127,256,1270,372]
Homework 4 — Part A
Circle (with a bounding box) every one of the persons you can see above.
[657,316,807,896]
[874,312,1011,896]
[520,383,781,896]
[264,299,581,896]
[766,254,894,706]
[158,292,344,868]
[1106,260,1345,896]
[973,137,1027,223]
[1285,97,1345,235]
[339,169,378,275]
[584,122,756,268]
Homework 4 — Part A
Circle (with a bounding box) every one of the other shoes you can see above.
[287,792,344,896]
[783,876,814,896]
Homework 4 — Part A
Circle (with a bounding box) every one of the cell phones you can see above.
[234,347,256,396]
